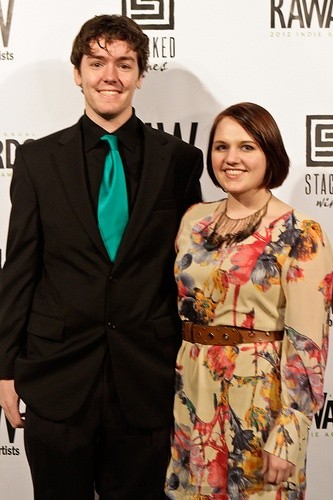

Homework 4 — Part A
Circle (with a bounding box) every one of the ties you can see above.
[98,134,129,262]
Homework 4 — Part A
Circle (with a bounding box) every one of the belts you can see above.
[181,321,284,346]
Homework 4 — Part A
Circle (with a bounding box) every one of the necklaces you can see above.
[207,190,272,249]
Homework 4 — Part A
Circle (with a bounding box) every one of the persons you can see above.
[1,14,203,500]
[164,101,333,500]
